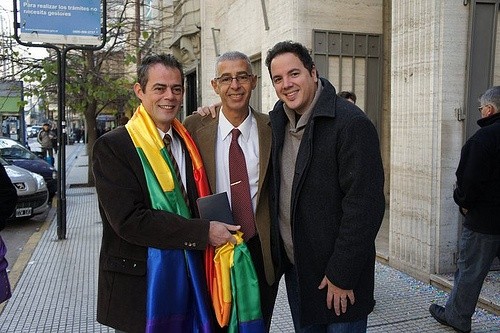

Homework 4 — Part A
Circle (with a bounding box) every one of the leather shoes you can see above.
[429,303,466,333]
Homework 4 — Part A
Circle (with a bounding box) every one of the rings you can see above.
[339,297,348,302]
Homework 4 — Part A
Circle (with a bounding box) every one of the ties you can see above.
[164,137,191,212]
[228,128,256,242]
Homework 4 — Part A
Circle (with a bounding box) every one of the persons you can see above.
[429,86,500,333]
[192,40,386,333]
[119,111,130,127]
[35,124,118,166]
[337,90,357,104]
[182,51,283,333]
[89,53,242,333]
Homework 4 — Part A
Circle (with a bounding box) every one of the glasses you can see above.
[215,74,254,84]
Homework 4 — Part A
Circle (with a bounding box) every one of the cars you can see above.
[0,136,58,199]
[0,156,50,220]
[26,127,39,138]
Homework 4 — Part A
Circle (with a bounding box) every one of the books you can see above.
[195,191,238,235]
